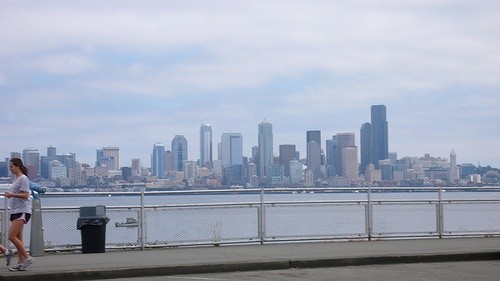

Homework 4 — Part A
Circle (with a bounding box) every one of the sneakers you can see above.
[8,262,23,271]
[5,249,15,266]
[19,255,35,271]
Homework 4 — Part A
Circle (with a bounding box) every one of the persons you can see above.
[0,245,15,266]
[5,158,34,269]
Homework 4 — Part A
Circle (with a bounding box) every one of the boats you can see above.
[115,218,139,227]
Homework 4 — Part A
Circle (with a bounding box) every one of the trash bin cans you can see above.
[77,206,110,253]
